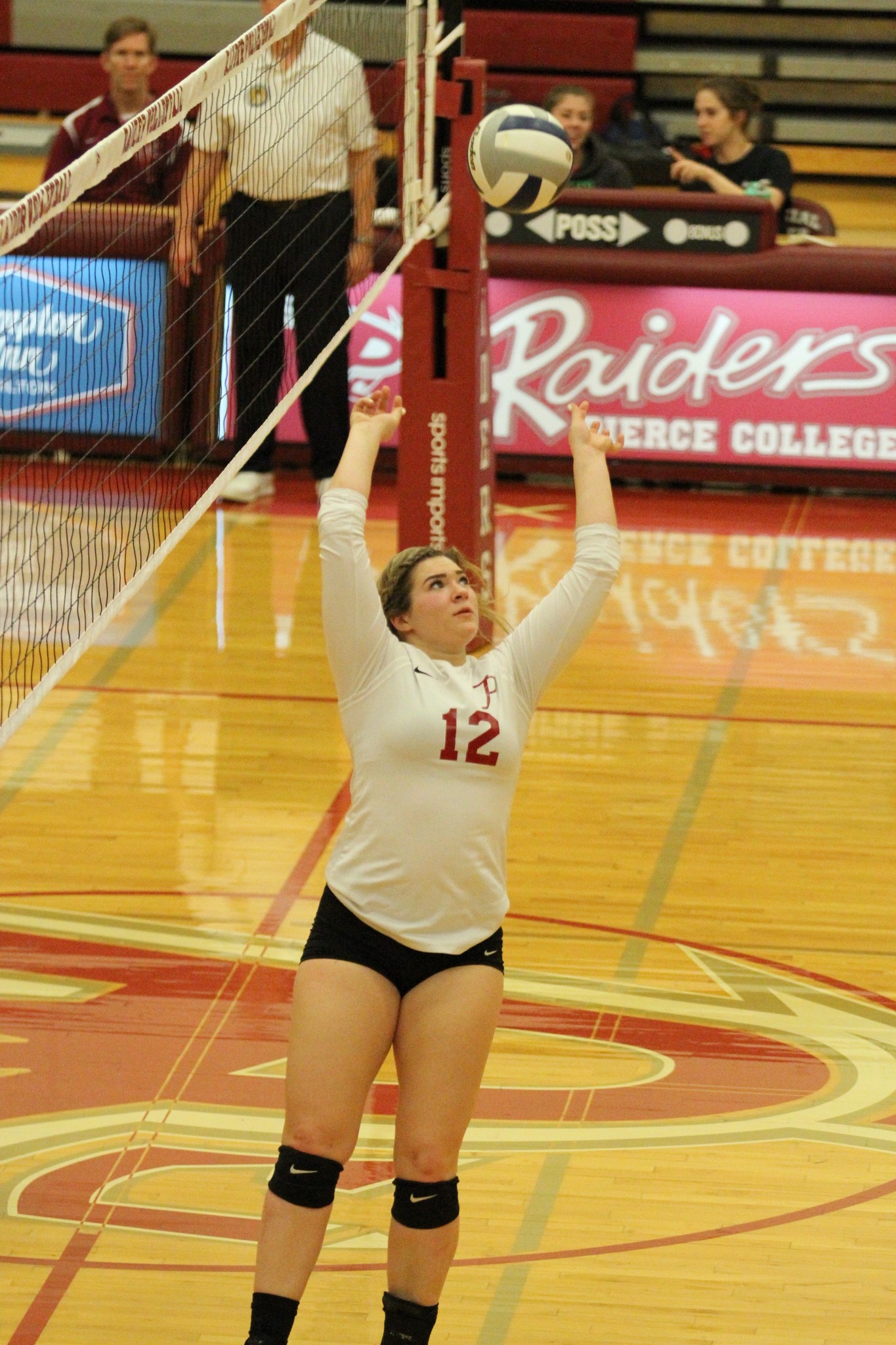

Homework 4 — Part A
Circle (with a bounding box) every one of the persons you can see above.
[243,387,622,1345]
[671,74,794,234]
[546,84,633,191]
[169,0,379,505]
[42,17,193,207]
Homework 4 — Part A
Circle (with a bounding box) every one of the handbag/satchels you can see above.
[599,93,673,183]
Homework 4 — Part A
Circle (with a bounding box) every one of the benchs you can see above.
[0,0,896,248]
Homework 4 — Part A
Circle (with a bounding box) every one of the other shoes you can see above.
[315,477,332,498]
[221,471,274,503]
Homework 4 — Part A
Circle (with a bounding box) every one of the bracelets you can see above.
[350,236,374,247]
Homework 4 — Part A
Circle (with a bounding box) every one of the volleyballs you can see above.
[465,103,574,215]
